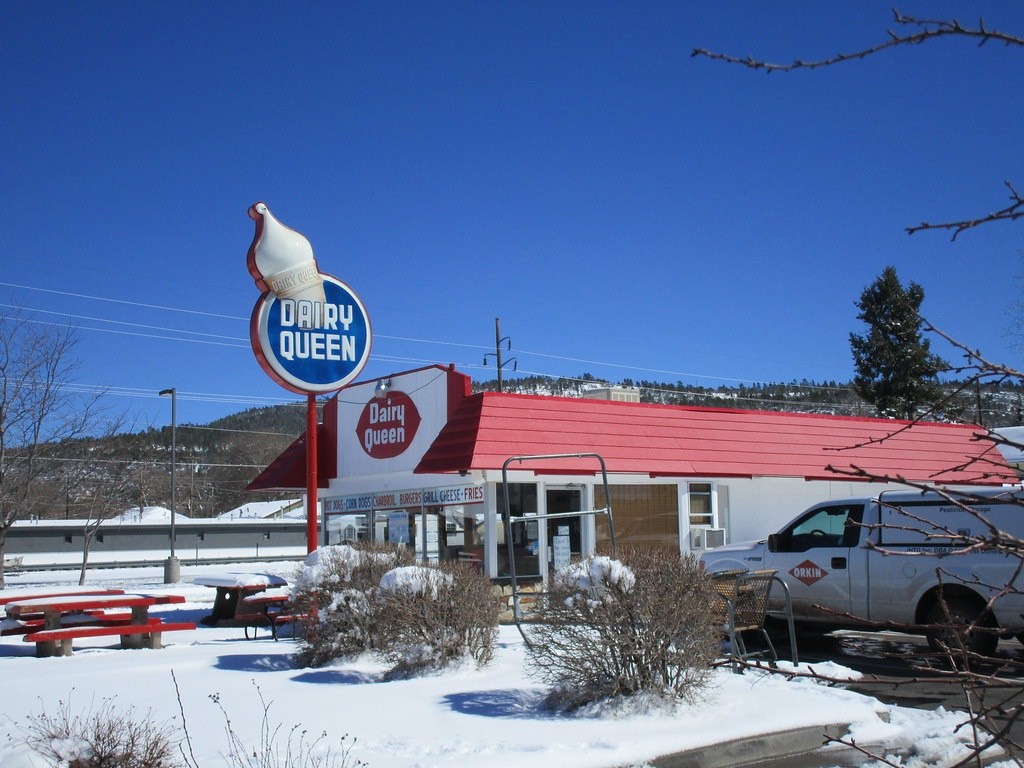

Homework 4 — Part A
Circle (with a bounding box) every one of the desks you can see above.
[0,587,125,614]
[4,593,186,658]
[243,595,310,640]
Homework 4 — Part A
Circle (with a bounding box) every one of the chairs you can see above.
[700,568,798,675]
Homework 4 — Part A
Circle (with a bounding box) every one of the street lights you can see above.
[158,388,181,583]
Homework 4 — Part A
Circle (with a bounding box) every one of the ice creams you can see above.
[247,202,330,331]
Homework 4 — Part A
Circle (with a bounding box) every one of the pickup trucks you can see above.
[696,486,1024,665]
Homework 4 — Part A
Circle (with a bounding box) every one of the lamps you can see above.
[374,379,391,398]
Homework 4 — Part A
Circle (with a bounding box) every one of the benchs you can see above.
[0,610,162,657]
[236,613,308,641]
[22,622,196,655]
